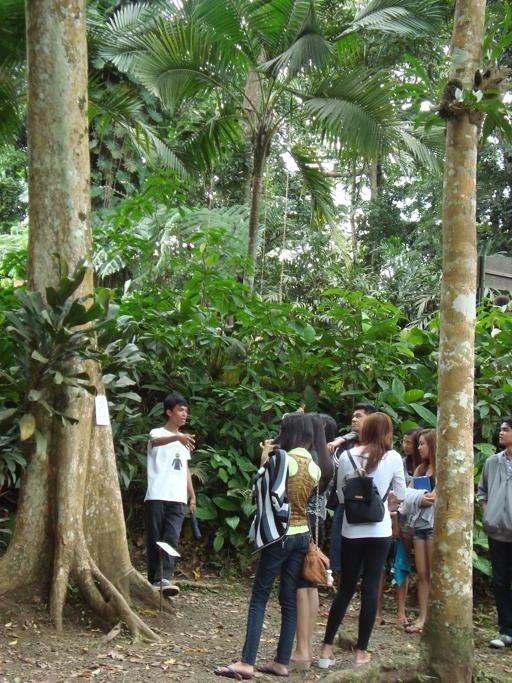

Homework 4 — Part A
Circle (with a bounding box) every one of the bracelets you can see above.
[391,510,400,516]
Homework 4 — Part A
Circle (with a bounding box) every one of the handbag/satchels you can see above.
[302,471,334,587]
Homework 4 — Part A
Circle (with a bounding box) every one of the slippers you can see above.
[405,624,424,633]
[310,660,314,666]
[354,654,371,667]
[214,665,254,680]
[257,665,289,677]
[396,618,408,624]
[318,653,336,669]
[376,617,385,625]
[288,661,311,670]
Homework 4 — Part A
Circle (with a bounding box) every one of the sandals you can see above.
[489,636,512,649]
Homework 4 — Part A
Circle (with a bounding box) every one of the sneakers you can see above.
[153,578,181,596]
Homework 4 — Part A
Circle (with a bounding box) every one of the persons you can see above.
[144,392,196,596]
[323,486,399,625]
[289,414,336,669]
[214,413,322,679]
[318,412,407,669]
[394,429,424,624]
[326,405,378,588]
[477,417,512,647]
[405,428,436,633]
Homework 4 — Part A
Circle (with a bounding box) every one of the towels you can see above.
[394,535,410,588]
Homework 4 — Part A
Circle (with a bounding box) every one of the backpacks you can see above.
[341,450,393,524]
